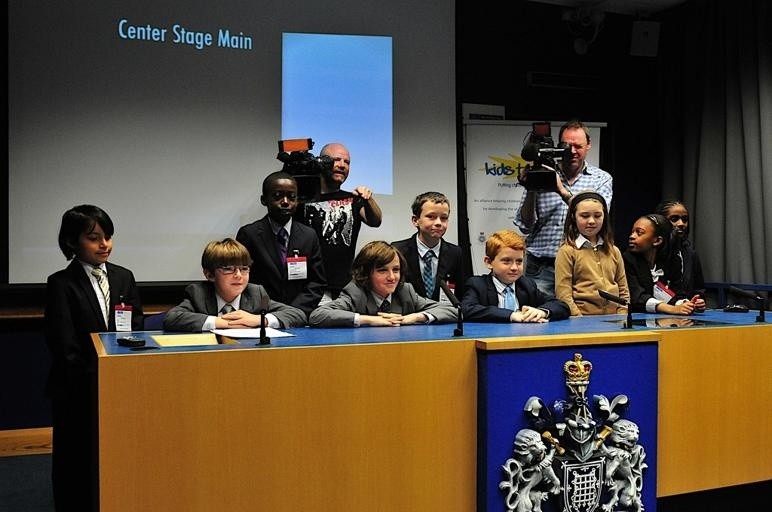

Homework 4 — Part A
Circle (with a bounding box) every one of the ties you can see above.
[422,251,435,297]
[276,230,290,263]
[91,267,111,319]
[222,305,235,315]
[504,288,516,312]
[380,299,392,312]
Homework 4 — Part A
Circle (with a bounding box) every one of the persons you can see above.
[307,242,463,328]
[39,204,143,511]
[235,173,328,316]
[555,190,632,314]
[163,239,308,330]
[327,206,347,243]
[652,194,707,302]
[460,231,573,324]
[512,121,612,293]
[619,212,706,316]
[292,143,384,296]
[387,190,473,301]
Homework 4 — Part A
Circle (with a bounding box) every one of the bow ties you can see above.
[650,267,664,283]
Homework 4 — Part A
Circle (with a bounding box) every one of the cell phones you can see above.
[117,336,145,347]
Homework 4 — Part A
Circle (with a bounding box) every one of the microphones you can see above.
[730,285,765,322]
[259,295,270,345]
[439,279,463,336]
[597,288,632,329]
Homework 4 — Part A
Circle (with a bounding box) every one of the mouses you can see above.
[724,304,749,312]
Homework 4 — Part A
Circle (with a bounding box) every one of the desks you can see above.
[87,300,770,511]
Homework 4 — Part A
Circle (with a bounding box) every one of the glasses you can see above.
[217,264,252,276]
